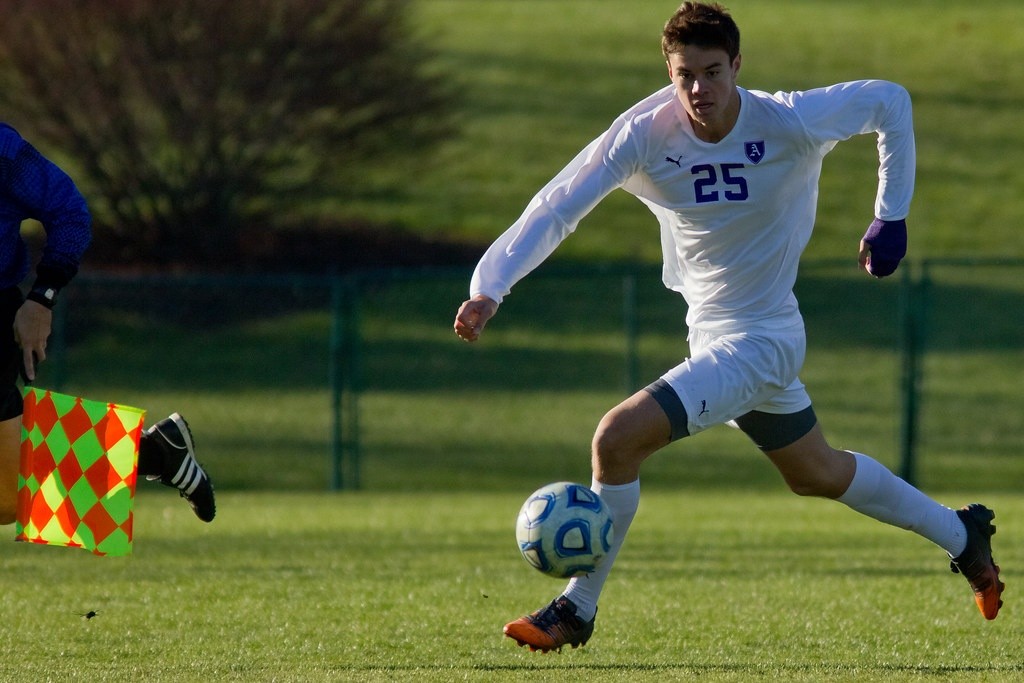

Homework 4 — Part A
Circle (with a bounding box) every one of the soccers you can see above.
[515,481,615,579]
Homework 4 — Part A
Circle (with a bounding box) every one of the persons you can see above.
[0,123,216,526]
[453,0,1005,652]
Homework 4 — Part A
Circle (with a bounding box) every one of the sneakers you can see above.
[503,595,599,655]
[142,410,217,522]
[946,503,1006,620]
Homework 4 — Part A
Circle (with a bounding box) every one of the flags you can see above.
[23,388,146,557]
